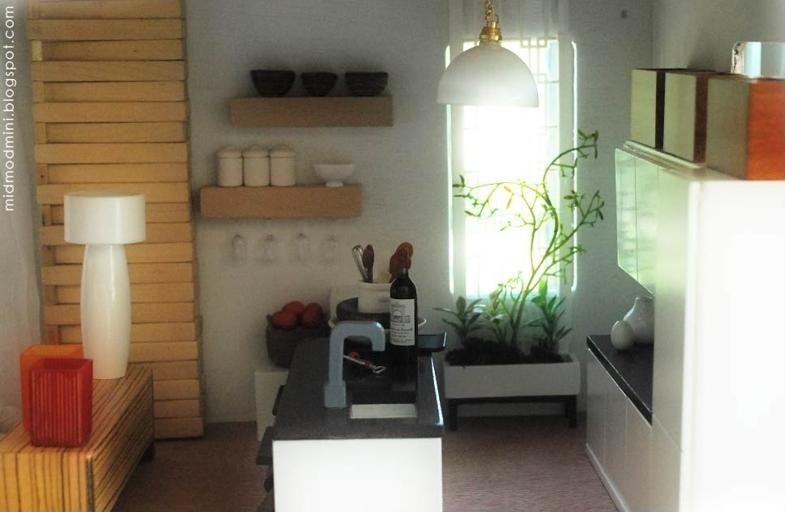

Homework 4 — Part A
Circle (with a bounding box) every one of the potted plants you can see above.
[433,128,603,399]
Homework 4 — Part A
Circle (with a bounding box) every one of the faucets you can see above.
[323,319,387,409]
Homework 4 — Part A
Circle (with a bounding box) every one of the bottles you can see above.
[242,145,270,187]
[215,145,243,186]
[388,257,418,347]
[270,144,299,187]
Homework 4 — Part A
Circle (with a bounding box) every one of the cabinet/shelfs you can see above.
[200,94,392,218]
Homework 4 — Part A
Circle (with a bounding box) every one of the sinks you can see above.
[347,389,419,421]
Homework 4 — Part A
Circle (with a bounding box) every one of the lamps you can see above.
[64,187,144,384]
[439,0,541,107]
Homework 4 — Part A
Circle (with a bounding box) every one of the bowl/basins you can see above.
[335,297,389,327]
[249,70,295,96]
[327,315,427,344]
[302,71,338,96]
[344,71,388,98]
[312,163,356,188]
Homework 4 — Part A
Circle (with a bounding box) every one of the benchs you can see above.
[0,363,154,512]
